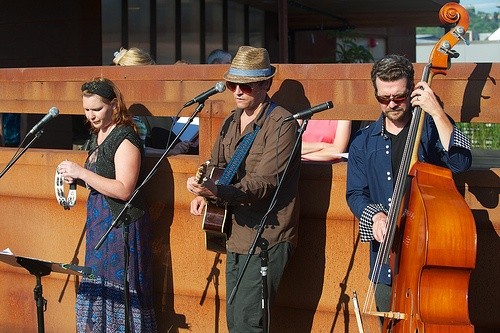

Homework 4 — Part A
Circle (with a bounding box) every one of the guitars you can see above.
[193,161,231,255]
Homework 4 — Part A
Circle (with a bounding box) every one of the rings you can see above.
[64,169,66,173]
[417,95,419,101]
[190,188,194,191]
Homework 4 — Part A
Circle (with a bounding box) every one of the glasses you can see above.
[375,89,411,104]
[225,81,255,96]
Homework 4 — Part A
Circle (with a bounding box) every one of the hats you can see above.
[222,45,277,84]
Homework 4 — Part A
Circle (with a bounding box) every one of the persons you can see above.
[296,120,352,163]
[110,47,236,155]
[0,113,22,147]
[346,54,472,333]
[57,77,149,333]
[186,45,302,333]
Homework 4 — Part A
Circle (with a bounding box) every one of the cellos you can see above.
[362,1,478,333]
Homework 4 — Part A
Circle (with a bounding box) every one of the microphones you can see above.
[284,100,334,121]
[29,106,60,135]
[183,81,226,108]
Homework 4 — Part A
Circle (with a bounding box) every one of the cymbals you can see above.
[53,161,79,210]
